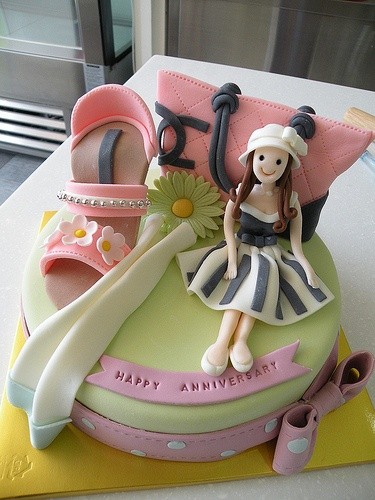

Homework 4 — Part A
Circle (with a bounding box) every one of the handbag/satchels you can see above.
[154,70,374,243]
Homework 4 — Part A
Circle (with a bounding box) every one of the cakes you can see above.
[21,143,343,461]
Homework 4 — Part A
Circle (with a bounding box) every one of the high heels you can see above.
[39,82,156,310]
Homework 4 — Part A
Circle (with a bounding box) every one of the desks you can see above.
[0,53,374,500]
